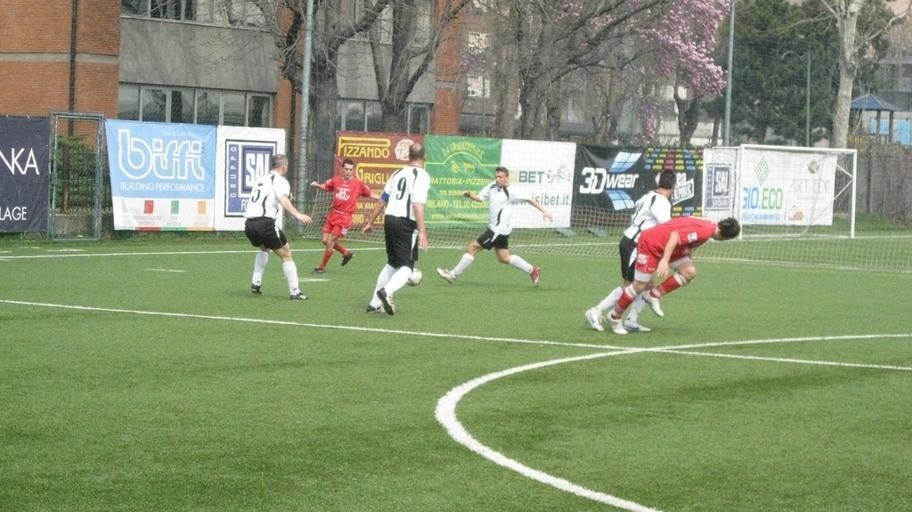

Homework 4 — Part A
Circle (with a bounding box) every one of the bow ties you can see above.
[527,264,544,290]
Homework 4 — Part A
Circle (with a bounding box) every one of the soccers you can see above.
[406,268,422,286]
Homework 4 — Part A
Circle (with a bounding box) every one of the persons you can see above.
[244,154,313,301]
[606,215,741,334]
[584,166,677,332]
[308,158,380,274]
[360,142,432,315]
[436,167,554,288]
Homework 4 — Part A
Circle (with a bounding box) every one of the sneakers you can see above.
[310,267,327,276]
[250,283,263,296]
[435,266,457,284]
[622,318,652,334]
[605,309,629,337]
[289,291,310,302]
[365,303,381,314]
[376,287,398,316]
[583,306,605,333]
[640,288,667,318]
[339,248,354,268]
